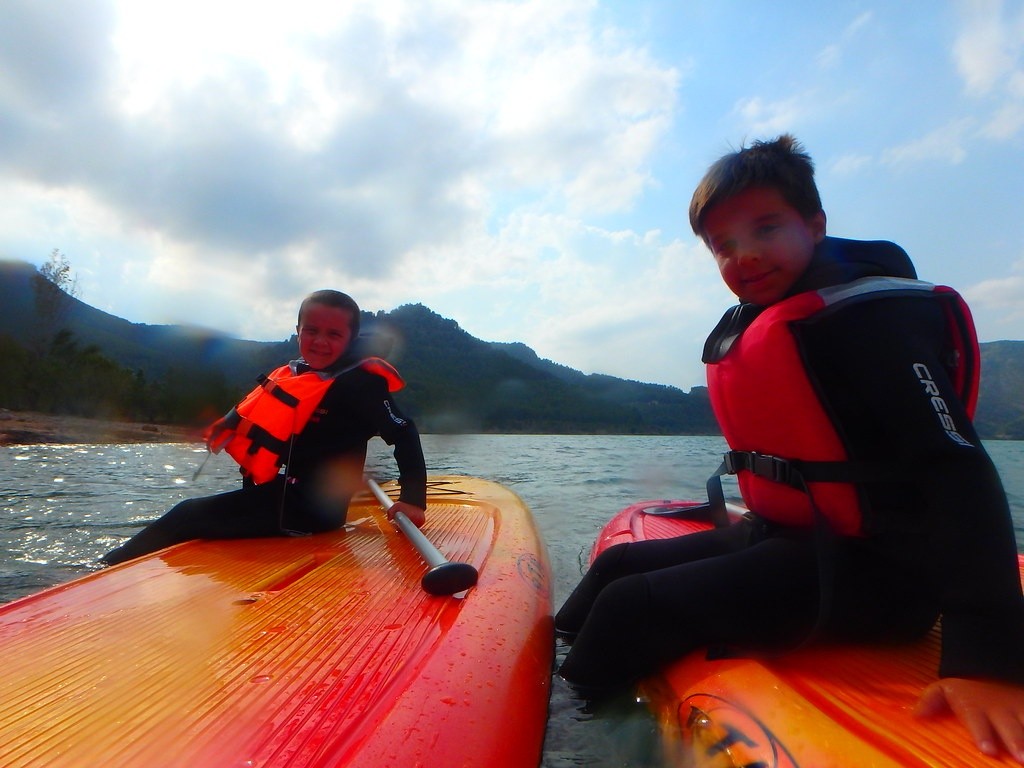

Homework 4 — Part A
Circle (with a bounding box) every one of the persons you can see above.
[99,288,429,569]
[550,134,1024,764]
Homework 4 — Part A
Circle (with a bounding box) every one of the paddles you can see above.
[364,473,475,598]
